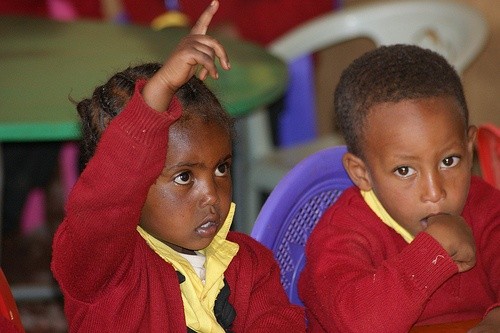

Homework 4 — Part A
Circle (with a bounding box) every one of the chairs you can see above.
[246,144,355,333]
[240,2,487,237]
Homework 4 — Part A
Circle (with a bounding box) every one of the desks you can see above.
[0,14,291,297]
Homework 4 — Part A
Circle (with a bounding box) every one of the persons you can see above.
[50,1,309,333]
[296,43,500,333]
